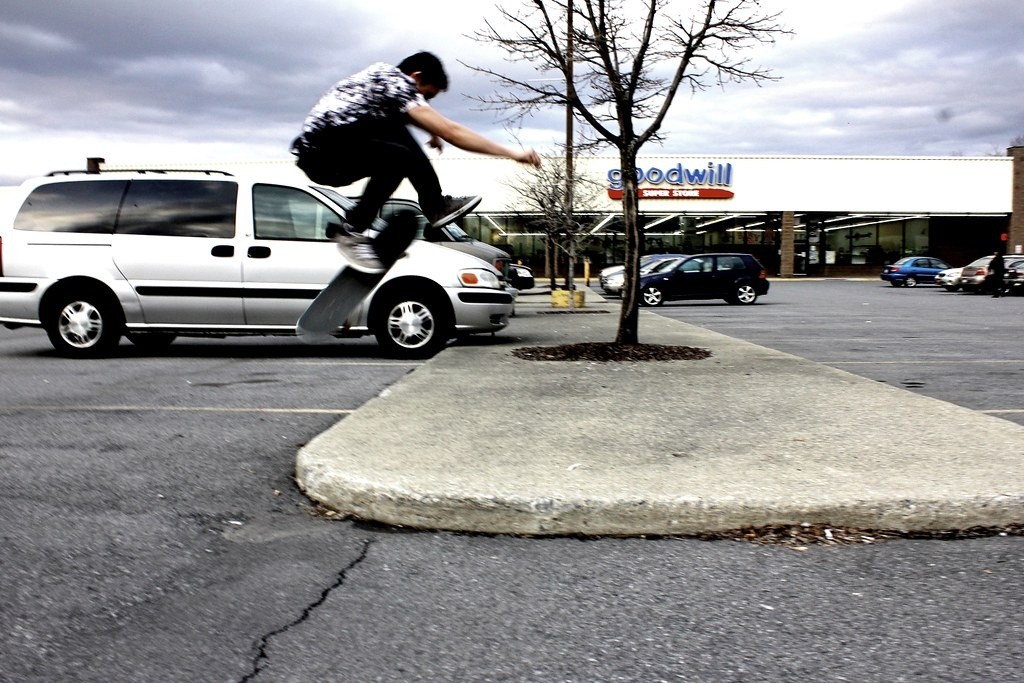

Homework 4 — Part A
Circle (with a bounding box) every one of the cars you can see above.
[599,254,731,298]
[934,253,1024,294]
[880,256,951,288]
[507,264,536,289]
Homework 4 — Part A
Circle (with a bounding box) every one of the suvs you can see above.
[637,253,770,307]
[0,168,514,358]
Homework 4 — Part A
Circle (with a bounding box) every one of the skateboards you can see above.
[293,210,421,345]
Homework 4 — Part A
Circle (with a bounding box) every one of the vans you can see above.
[346,196,512,277]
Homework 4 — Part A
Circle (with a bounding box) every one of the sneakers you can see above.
[430,195,483,228]
[336,236,387,273]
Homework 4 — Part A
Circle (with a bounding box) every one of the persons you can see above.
[988,249,1006,298]
[291,51,545,275]
